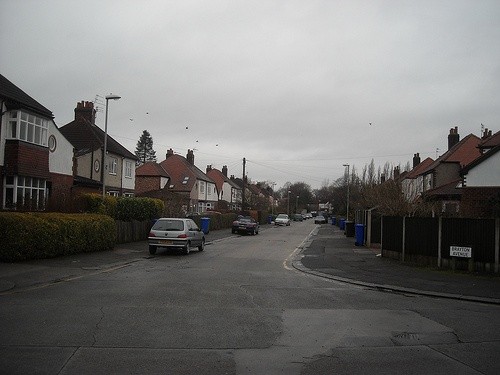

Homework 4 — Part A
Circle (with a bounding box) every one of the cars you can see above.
[315,216,326,224]
[274,214,290,226]
[292,213,312,221]
[148,217,205,255]
[232,216,260,235]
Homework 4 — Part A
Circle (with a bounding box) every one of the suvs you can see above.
[311,210,318,216]
[316,211,328,222]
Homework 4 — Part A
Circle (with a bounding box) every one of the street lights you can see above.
[287,191,292,215]
[296,196,299,214]
[272,183,277,218]
[99,94,121,197]
[342,164,350,220]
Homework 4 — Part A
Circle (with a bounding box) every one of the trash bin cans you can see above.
[267,215,272,224]
[346,221,355,237]
[152,219,157,225]
[339,218,345,230]
[200,217,211,234]
[354,224,367,246]
[332,217,336,225]
